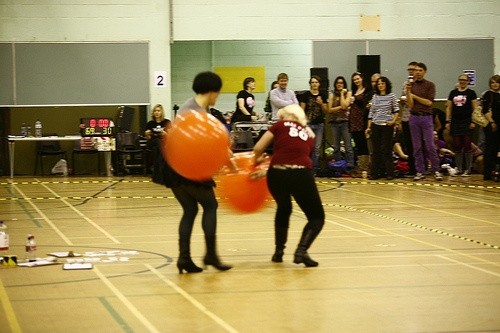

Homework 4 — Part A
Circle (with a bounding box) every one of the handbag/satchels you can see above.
[473,104,493,127]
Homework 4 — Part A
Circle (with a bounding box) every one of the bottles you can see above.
[25,234,37,262]
[0,221,8,257]
[34,118,41,137]
[27,125,31,138]
[21,123,26,137]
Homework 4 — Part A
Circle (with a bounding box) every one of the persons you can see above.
[363,72,409,170]
[151,72,235,274]
[482,75,500,181]
[246,103,326,265]
[365,77,399,180]
[269,73,299,123]
[230,76,262,133]
[264,81,278,122]
[298,75,327,174]
[405,63,444,180]
[443,74,480,176]
[398,61,423,176]
[326,76,355,172]
[431,107,446,136]
[345,72,371,167]
[143,103,173,181]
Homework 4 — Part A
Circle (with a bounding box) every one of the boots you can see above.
[294,228,320,267]
[177,235,203,274]
[271,227,288,262]
[202,232,233,271]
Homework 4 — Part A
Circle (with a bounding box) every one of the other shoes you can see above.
[369,159,500,184]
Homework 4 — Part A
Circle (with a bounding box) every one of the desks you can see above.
[7,133,117,180]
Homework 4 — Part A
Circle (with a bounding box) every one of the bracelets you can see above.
[445,120,451,123]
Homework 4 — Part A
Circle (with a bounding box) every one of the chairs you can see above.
[113,131,147,178]
[35,133,69,179]
[69,142,102,177]
